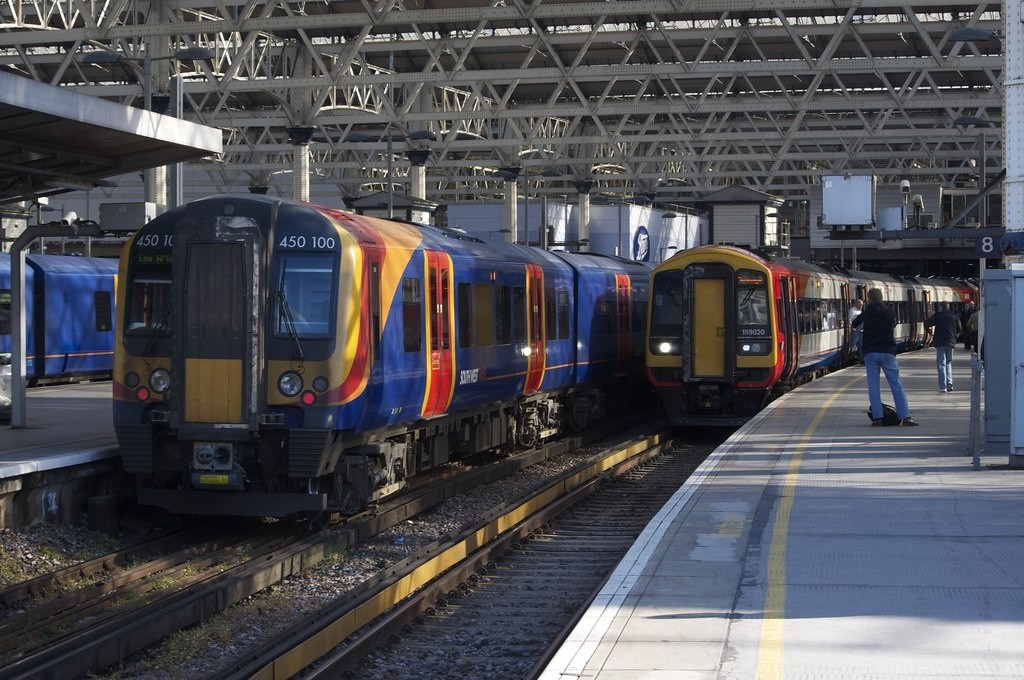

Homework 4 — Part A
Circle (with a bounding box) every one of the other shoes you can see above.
[860,361,865,366]
[974,351,977,355]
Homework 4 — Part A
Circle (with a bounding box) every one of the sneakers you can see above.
[938,387,945,392]
[947,382,954,391]
[871,419,880,424]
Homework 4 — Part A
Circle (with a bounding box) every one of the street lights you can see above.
[489,229,513,241]
[659,246,677,264]
[82,46,217,201]
[659,211,689,250]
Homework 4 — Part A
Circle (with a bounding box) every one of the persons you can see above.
[924,300,963,393]
[852,288,920,426]
[848,299,865,365]
[959,301,980,353]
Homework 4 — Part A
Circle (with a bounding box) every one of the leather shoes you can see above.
[903,418,919,427]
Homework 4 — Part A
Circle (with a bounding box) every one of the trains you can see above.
[0,249,120,389]
[110,196,649,523]
[638,244,980,434]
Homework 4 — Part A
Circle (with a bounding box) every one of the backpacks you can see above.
[867,403,901,426]
